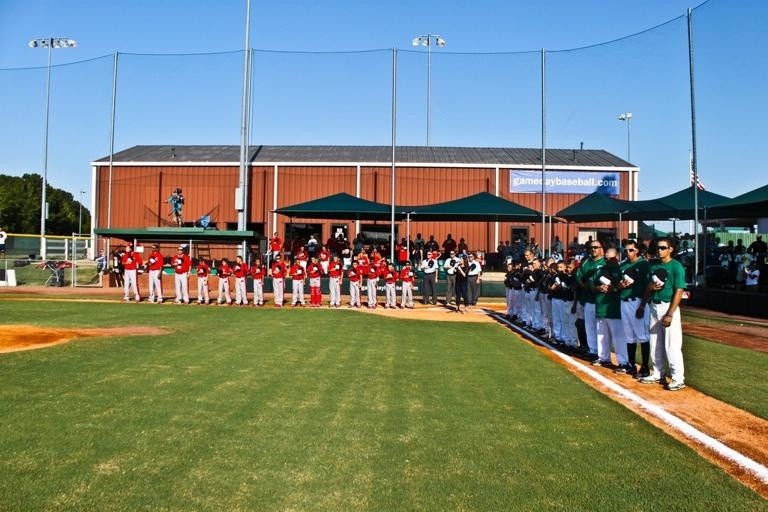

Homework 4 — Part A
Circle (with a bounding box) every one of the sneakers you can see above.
[584,350,612,366]
[616,363,666,383]
[667,380,685,390]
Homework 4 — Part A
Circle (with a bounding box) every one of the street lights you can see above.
[26,38,77,258]
[616,111,635,167]
[79,190,86,237]
[409,33,449,149]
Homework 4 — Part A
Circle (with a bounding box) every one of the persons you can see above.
[164,187,185,228]
[0,226,7,261]
[498,232,767,391]
[94,232,486,309]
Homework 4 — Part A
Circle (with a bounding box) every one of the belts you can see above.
[647,299,661,304]
[622,297,636,302]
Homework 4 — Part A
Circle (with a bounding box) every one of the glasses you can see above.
[589,245,603,249]
[624,248,636,252]
[656,245,672,250]
